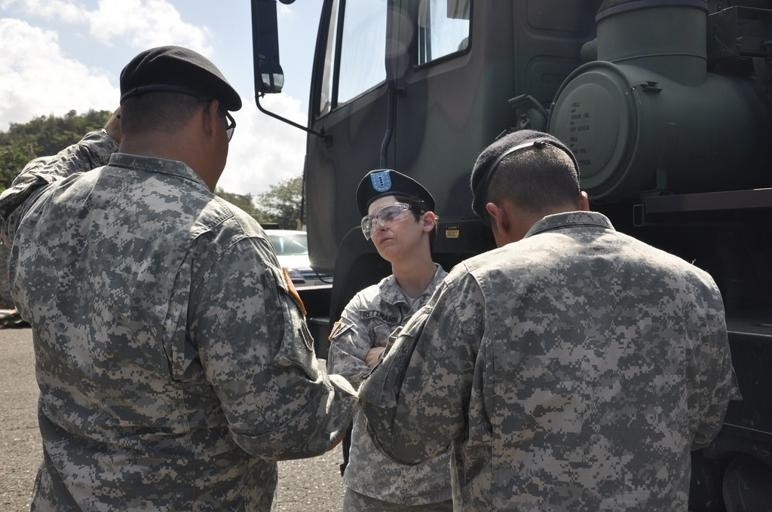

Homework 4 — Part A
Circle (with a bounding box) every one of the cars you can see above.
[264,230,313,279]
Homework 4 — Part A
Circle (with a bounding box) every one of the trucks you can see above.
[251,0,772,512]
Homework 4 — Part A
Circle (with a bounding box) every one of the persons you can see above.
[327,168,462,510]
[358,127,743,509]
[3,45,356,509]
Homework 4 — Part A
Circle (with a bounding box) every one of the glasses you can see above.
[218,98,236,143]
[358,202,429,242]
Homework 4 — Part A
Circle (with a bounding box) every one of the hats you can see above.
[354,166,436,215]
[117,45,243,113]
[469,127,581,238]
